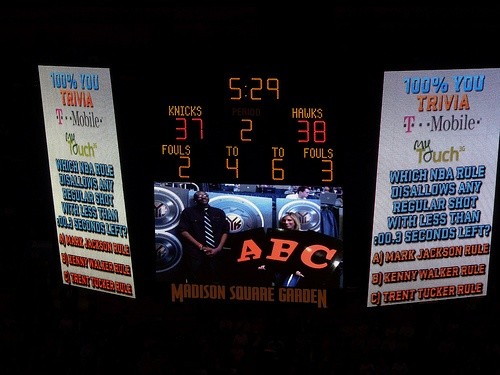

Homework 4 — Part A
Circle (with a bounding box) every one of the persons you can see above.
[279,212,302,231]
[324,186,334,193]
[286,186,310,199]
[176,191,229,257]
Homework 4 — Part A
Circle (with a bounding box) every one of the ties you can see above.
[203,208,215,249]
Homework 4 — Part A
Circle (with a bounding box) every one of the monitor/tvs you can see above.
[146,180,349,319]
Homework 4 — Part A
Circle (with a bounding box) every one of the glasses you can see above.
[196,194,209,198]
